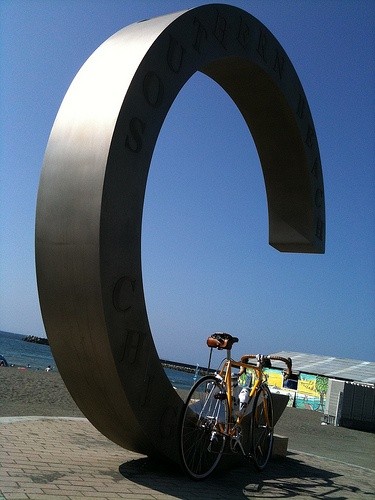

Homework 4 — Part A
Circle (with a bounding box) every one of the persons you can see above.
[0,353,51,373]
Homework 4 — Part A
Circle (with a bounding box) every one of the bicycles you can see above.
[176,332,293,481]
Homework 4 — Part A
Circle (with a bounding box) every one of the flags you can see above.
[193,363,200,381]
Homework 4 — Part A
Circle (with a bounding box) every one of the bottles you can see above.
[238,386,251,414]
